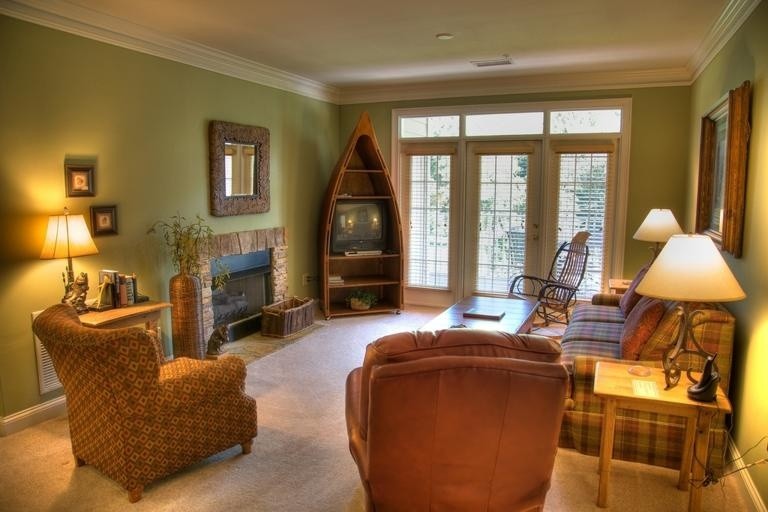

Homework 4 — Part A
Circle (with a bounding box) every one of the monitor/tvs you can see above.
[331,200,395,252]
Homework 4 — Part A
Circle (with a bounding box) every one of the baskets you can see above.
[261,294,314,338]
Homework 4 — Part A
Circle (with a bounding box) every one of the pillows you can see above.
[638,300,685,361]
[619,264,650,320]
[620,295,665,361]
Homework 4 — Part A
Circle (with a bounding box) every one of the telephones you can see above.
[688,352,719,401]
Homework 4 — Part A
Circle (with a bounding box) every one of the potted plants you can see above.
[344,287,379,311]
[147,209,231,360]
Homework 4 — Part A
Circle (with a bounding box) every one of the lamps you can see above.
[633,208,684,251]
[39,206,98,304]
[634,232,746,390]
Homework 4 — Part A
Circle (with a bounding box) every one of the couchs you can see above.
[557,293,737,479]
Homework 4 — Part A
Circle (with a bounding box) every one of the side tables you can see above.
[78,294,173,335]
[608,279,634,294]
[592,361,732,512]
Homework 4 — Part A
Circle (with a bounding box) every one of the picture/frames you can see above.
[90,205,118,237]
[64,163,95,197]
[694,80,751,258]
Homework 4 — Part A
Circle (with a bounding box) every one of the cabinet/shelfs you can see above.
[318,111,404,321]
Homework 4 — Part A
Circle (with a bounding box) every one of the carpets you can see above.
[223,323,325,366]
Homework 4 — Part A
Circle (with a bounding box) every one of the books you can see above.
[463,308,505,320]
[330,275,344,285]
[99,270,138,308]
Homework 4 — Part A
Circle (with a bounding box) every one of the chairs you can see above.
[507,231,593,326]
[344,329,570,511]
[31,304,258,504]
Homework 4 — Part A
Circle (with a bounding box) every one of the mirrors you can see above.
[209,120,270,217]
